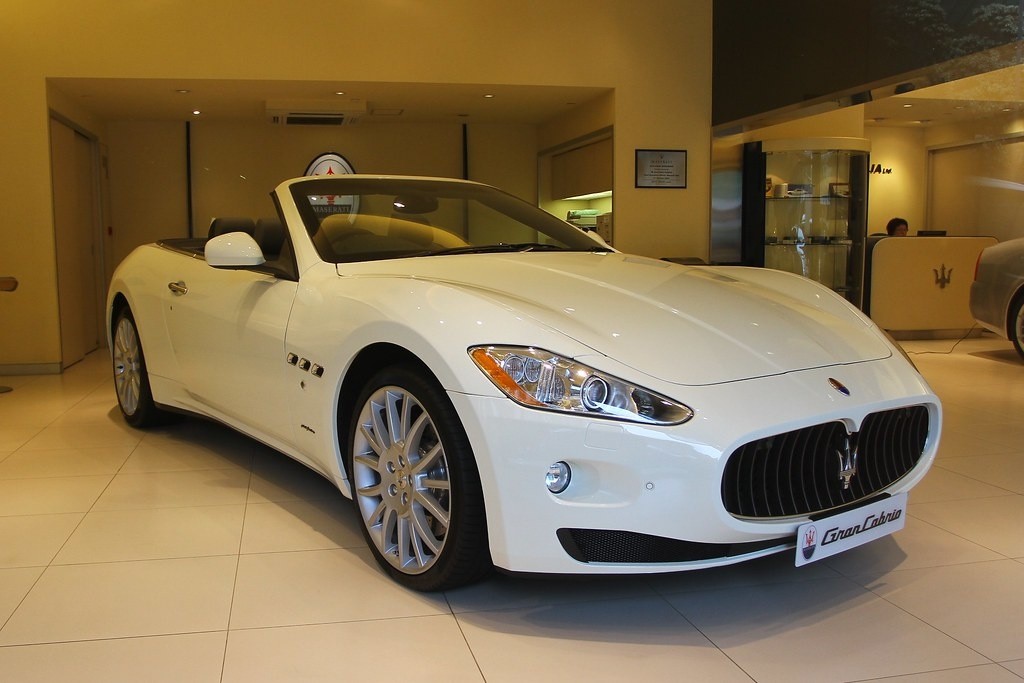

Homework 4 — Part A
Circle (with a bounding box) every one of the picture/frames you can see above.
[635,148,687,188]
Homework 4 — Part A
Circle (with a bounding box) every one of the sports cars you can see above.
[103,173,944,594]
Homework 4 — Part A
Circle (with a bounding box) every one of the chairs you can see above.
[390,213,435,248]
[208,217,256,262]
[253,218,284,262]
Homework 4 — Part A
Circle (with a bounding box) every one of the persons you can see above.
[887,218,908,237]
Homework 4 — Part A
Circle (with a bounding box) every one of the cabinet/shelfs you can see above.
[742,136,872,314]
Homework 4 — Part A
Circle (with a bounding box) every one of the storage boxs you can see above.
[774,183,813,198]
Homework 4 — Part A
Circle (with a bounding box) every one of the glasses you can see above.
[897,228,909,232]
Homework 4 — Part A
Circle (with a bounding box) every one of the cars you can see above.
[969,236,1024,361]
